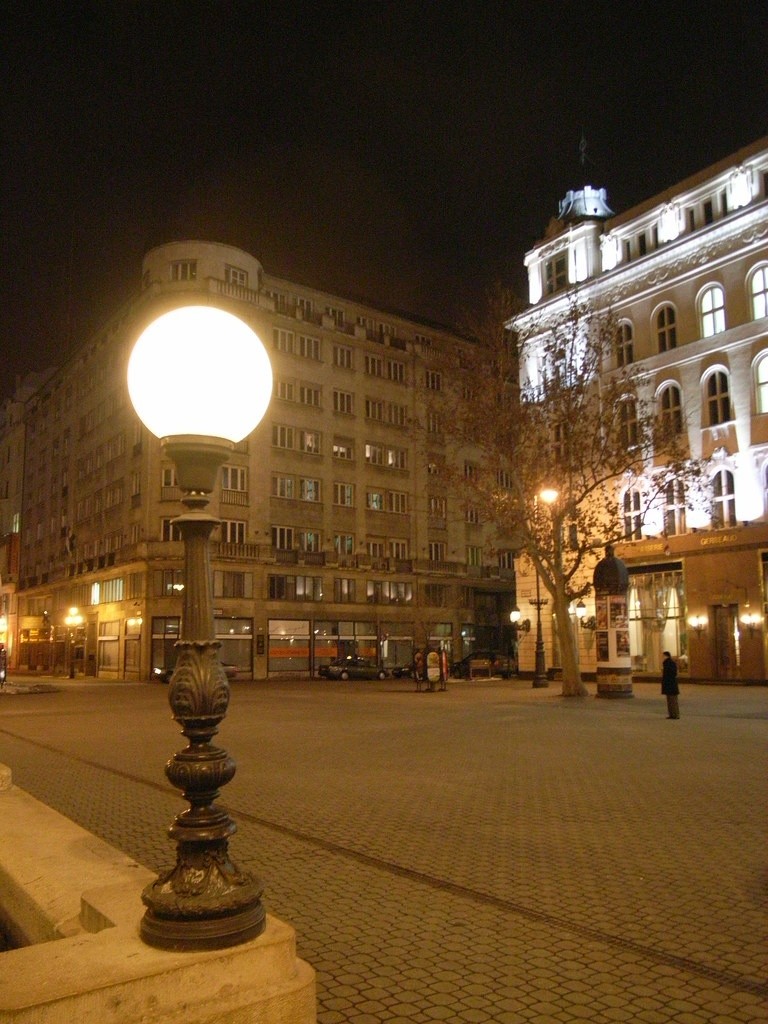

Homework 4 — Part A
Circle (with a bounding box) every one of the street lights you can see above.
[530,486,560,687]
[63,607,83,678]
[124,304,278,954]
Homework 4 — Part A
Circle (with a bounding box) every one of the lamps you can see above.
[510,606,530,632]
[740,613,762,638]
[689,615,708,640]
[576,599,596,630]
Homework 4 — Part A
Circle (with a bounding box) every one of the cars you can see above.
[152,658,241,685]
[450,649,513,680]
[392,661,416,680]
[317,657,390,681]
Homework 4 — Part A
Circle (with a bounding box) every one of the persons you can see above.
[661,652,680,720]
